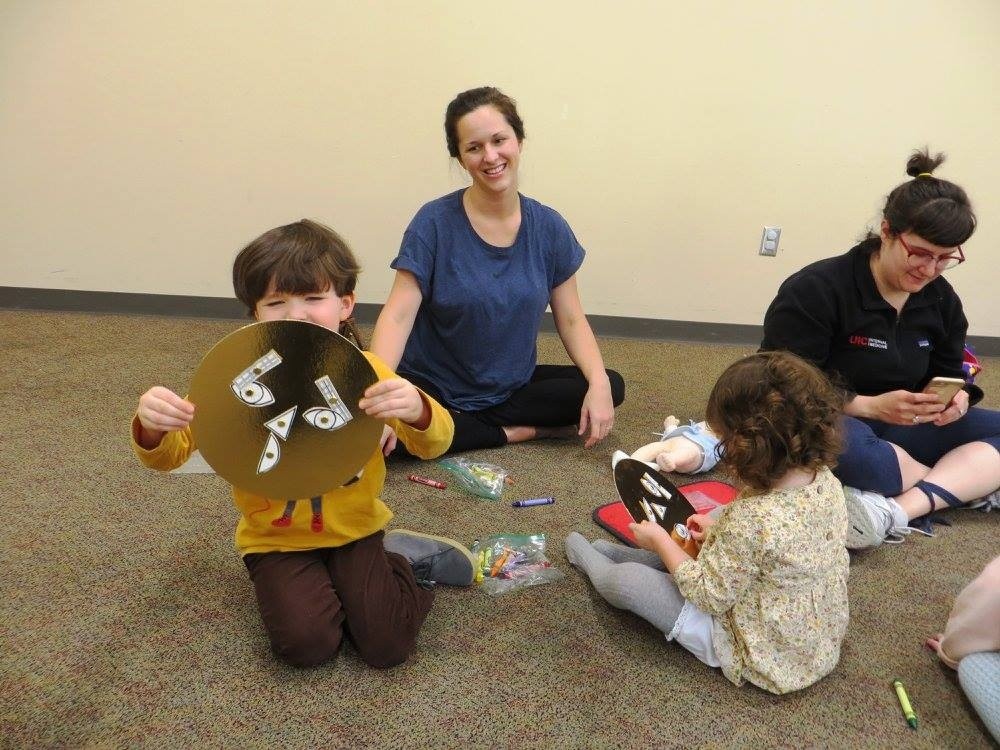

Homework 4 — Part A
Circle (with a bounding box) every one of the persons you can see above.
[611,414,724,474]
[368,87,626,463]
[565,349,851,695]
[129,218,477,669]
[927,552,1000,741]
[756,152,1000,550]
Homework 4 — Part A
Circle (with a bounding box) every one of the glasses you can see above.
[891,227,966,272]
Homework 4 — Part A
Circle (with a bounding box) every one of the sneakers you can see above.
[843,484,939,550]
[958,489,1000,514]
[385,529,475,589]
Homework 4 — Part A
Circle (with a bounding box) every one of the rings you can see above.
[959,410,963,415]
[912,415,919,424]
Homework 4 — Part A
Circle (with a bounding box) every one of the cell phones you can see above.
[916,377,965,411]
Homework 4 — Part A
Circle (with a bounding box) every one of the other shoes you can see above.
[531,424,580,442]
